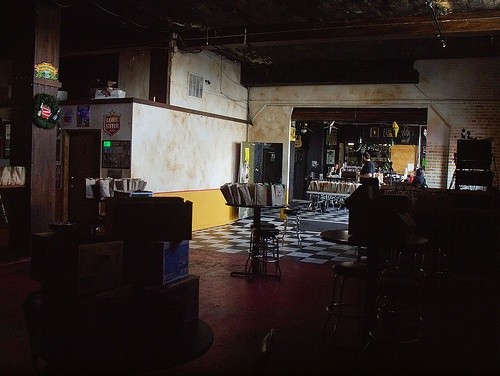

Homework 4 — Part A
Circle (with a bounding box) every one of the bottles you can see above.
[50,108,62,124]
[37,103,43,118]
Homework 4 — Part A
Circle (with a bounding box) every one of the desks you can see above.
[227,200,285,275]
[322,229,429,249]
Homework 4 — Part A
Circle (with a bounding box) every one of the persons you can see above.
[411,169,426,189]
[359,153,374,184]
[330,164,340,175]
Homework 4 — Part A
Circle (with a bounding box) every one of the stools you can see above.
[323,261,367,345]
[245,222,282,282]
[281,208,303,251]
[364,276,426,372]
